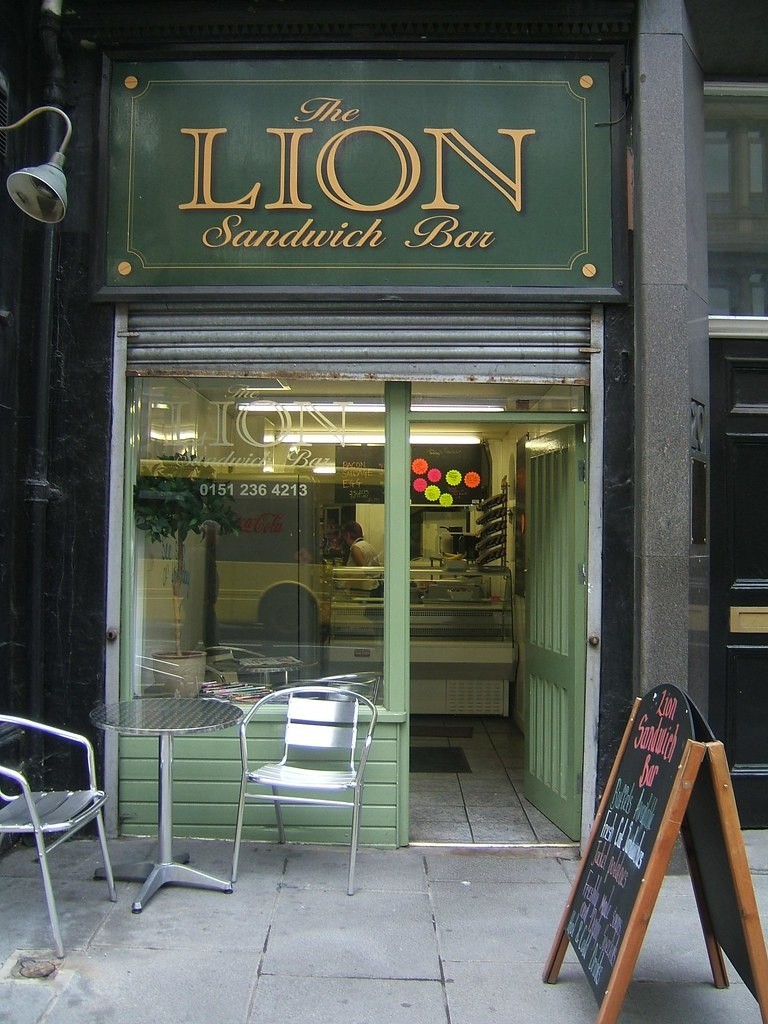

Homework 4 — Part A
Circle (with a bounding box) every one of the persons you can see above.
[342,521,381,601]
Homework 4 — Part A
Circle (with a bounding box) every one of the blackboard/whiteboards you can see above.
[567,684,762,1010]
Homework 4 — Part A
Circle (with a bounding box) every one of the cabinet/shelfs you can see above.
[329,565,515,650]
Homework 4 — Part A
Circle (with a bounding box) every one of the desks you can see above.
[211,654,318,690]
[87,696,245,915]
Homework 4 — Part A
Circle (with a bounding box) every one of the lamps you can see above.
[262,431,486,444]
[232,398,509,415]
[0,105,73,224]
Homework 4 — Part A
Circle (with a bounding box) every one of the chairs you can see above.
[290,670,379,706]
[257,581,322,663]
[201,645,268,690]
[230,685,378,896]
[0,714,121,959]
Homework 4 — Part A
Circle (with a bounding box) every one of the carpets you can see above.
[409,725,475,739]
[409,746,473,774]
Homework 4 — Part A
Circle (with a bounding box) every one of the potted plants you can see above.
[130,447,243,699]
